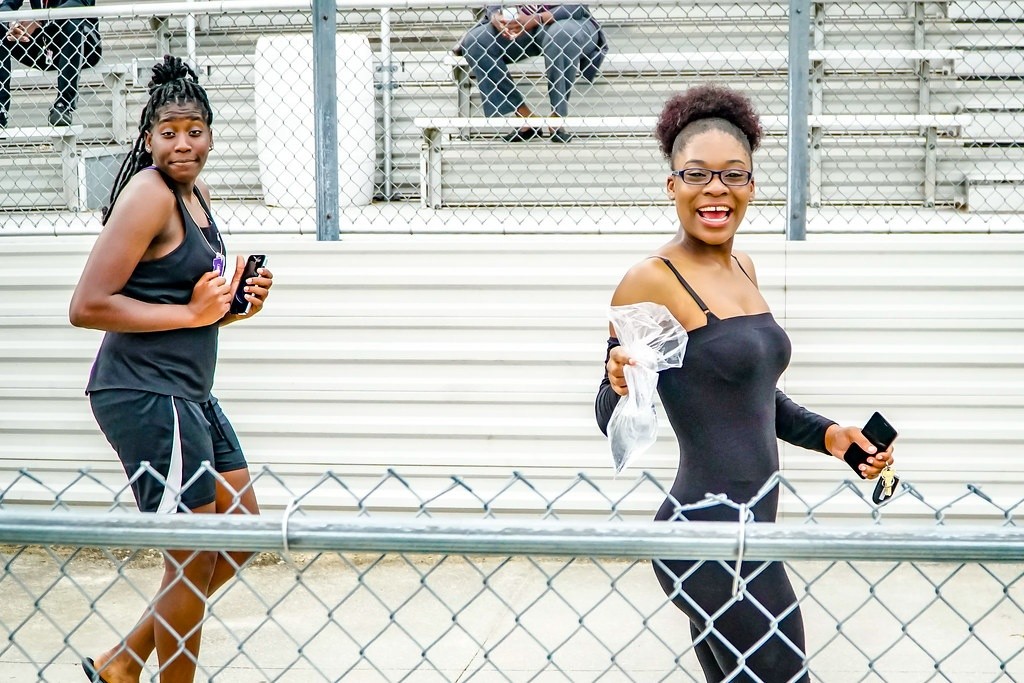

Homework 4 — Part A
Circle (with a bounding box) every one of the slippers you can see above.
[82,657,107,683]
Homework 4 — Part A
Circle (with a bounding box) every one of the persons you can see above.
[0,0,102,128]
[68,55,273,683]
[595,85,894,683]
[453,5,609,143]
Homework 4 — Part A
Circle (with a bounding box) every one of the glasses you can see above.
[670,160,766,187]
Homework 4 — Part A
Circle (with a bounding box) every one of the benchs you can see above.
[470,0,957,49]
[0,124,85,211]
[447,49,963,114]
[413,112,972,209]
[10,63,133,145]
[95,13,172,58]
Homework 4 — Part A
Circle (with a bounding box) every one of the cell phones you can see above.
[230,254,268,315]
[502,8,518,19]
[843,412,898,479]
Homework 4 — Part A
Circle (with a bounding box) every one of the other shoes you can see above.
[0,112,8,128]
[503,127,543,143]
[550,132,572,142]
[47,106,72,126]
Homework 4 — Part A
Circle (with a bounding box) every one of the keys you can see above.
[873,460,899,505]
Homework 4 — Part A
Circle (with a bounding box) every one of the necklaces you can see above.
[152,164,225,268]
